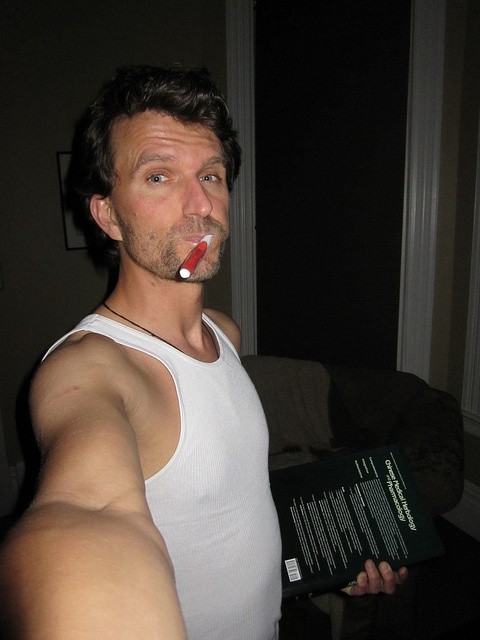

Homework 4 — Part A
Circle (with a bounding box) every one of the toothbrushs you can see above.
[179,235,213,280]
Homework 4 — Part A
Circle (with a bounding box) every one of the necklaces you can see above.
[100,303,220,368]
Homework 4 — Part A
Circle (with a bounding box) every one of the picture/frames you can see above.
[59,149,100,252]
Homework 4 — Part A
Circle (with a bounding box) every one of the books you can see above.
[270,447,445,606]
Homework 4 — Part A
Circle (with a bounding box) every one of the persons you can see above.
[0,63,412,639]
